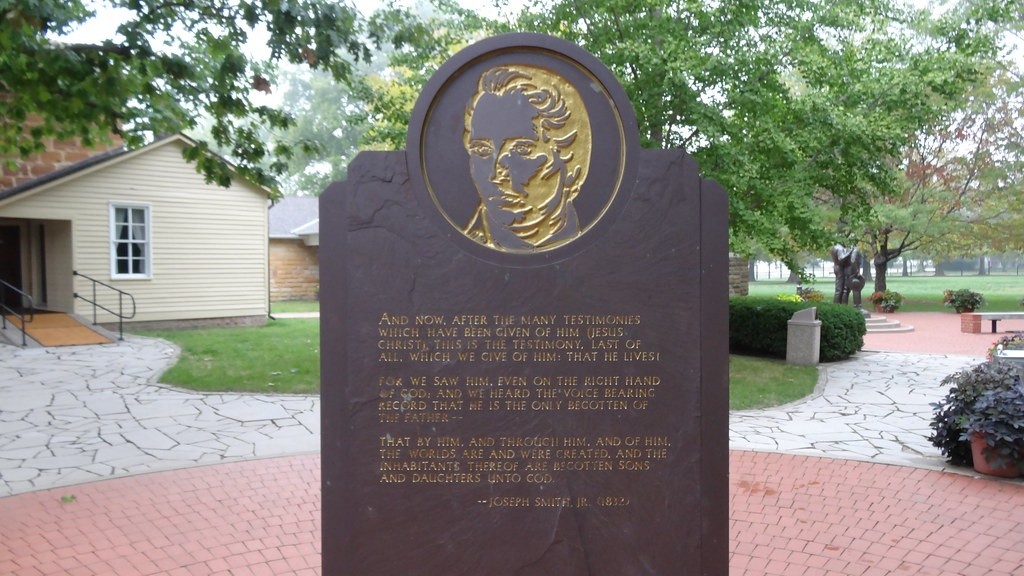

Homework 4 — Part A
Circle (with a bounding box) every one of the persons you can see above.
[831,234,865,310]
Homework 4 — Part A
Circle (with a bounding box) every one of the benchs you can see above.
[961,312,1024,333]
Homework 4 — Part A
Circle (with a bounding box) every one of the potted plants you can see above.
[928,358,1024,477]
[868,289,906,313]
[943,289,985,313]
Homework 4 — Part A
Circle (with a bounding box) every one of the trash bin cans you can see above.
[786,307,823,367]
